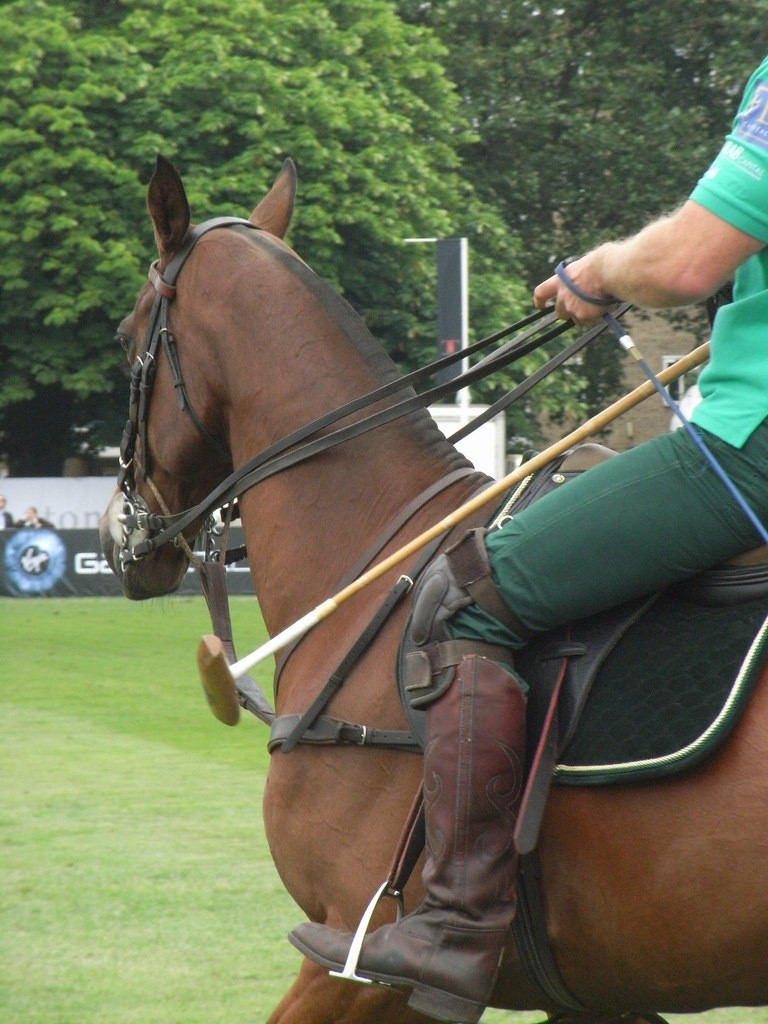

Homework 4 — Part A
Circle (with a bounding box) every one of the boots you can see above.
[289,655,528,1024]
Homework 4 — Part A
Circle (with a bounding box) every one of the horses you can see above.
[97,150,768,1024]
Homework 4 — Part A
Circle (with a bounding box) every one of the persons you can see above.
[16,507,53,529]
[284,54,768,1024]
[0,496,14,527]
